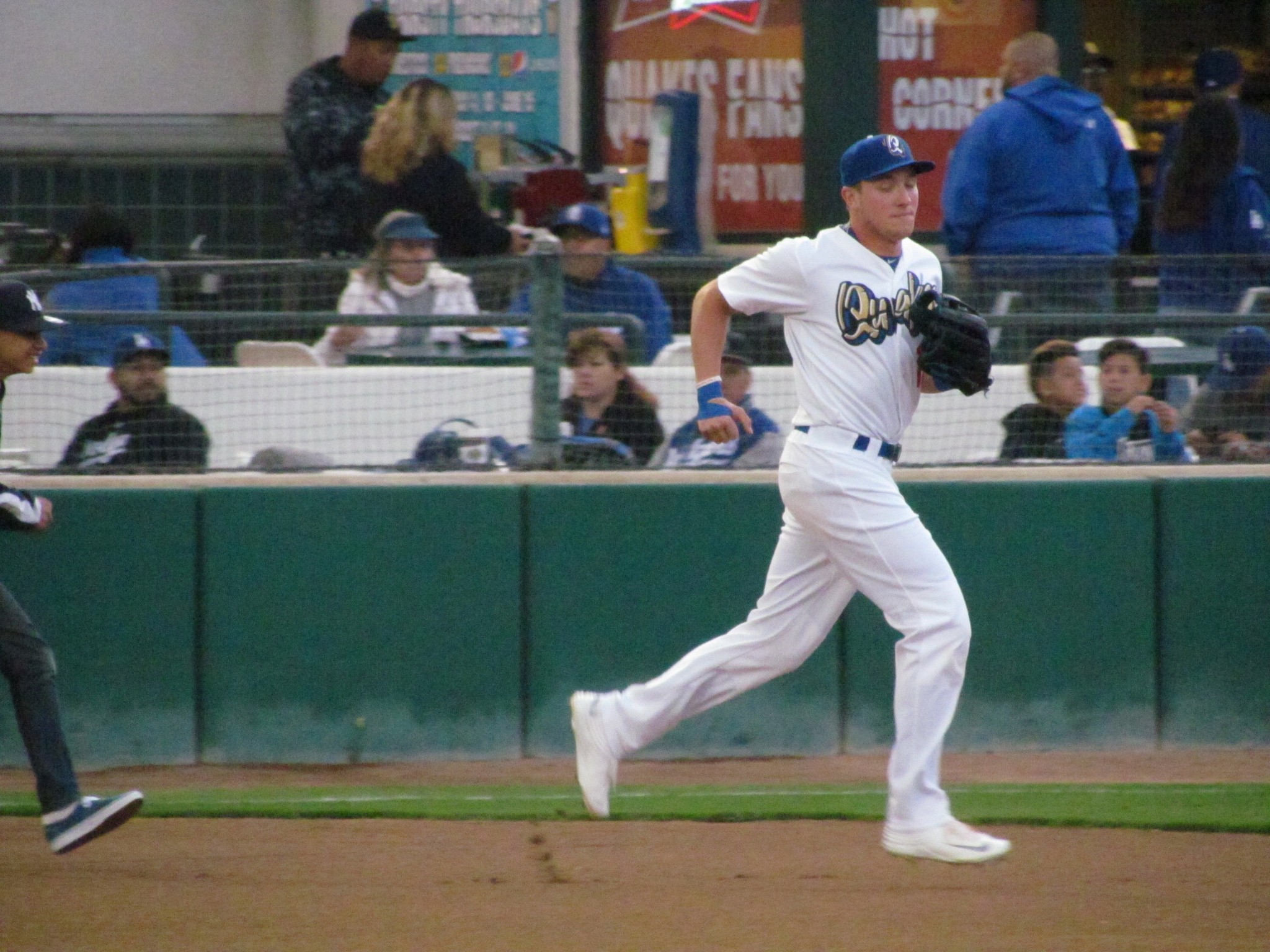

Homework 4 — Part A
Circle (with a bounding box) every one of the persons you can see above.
[557,327,665,471]
[1,280,143,858]
[37,205,208,369]
[281,8,531,260]
[503,198,672,363]
[999,338,1192,467]
[646,331,787,470]
[568,137,1014,863]
[938,29,1142,342]
[311,209,480,364]
[57,335,212,469]
[1178,326,1270,462]
[1152,43,1269,341]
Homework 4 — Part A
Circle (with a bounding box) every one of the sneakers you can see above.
[43,791,145,857]
[880,818,1009,865]
[570,686,626,819]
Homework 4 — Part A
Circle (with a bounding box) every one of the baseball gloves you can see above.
[908,290,993,397]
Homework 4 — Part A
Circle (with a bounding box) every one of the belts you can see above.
[794,422,902,463]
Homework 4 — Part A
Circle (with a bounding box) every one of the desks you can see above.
[1079,344,1221,377]
[342,334,534,365]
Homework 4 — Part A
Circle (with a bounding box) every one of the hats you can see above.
[840,133,934,187]
[718,330,754,365]
[1193,47,1242,93]
[1204,326,1269,391]
[0,278,68,335]
[350,9,416,42]
[111,332,168,365]
[379,215,439,242]
[549,203,613,237]
